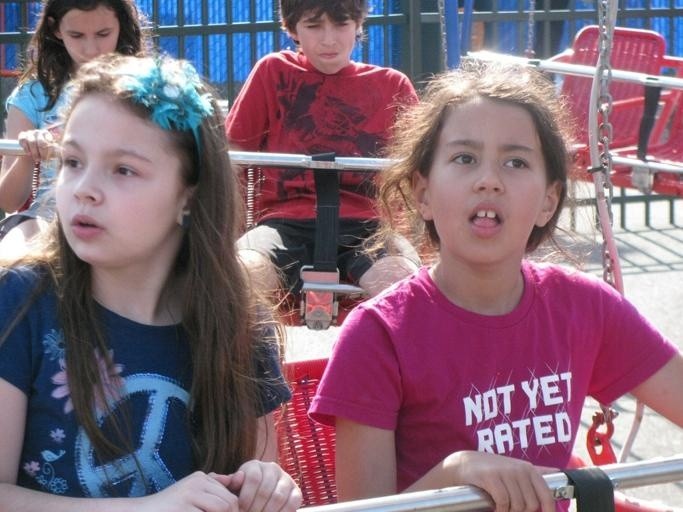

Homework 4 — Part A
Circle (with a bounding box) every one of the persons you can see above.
[0,46,311,510]
[305,57,682,512]
[0,2,163,270]
[217,0,426,316]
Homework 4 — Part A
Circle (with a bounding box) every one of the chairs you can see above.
[537,24,683,233]
